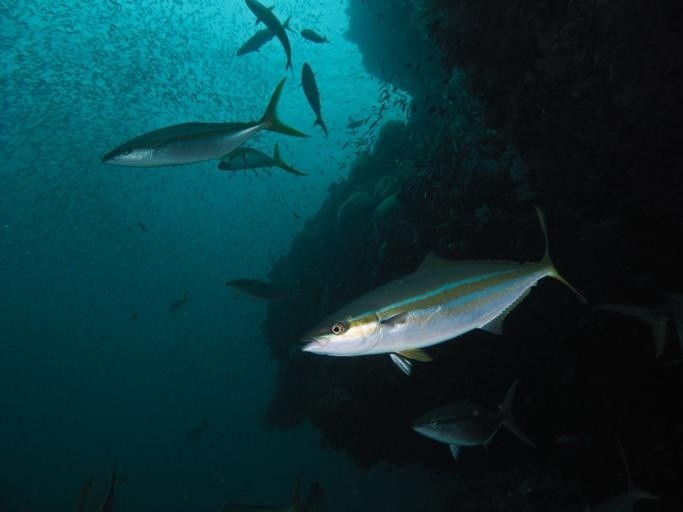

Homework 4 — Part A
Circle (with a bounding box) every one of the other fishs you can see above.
[0,1,459,178]
